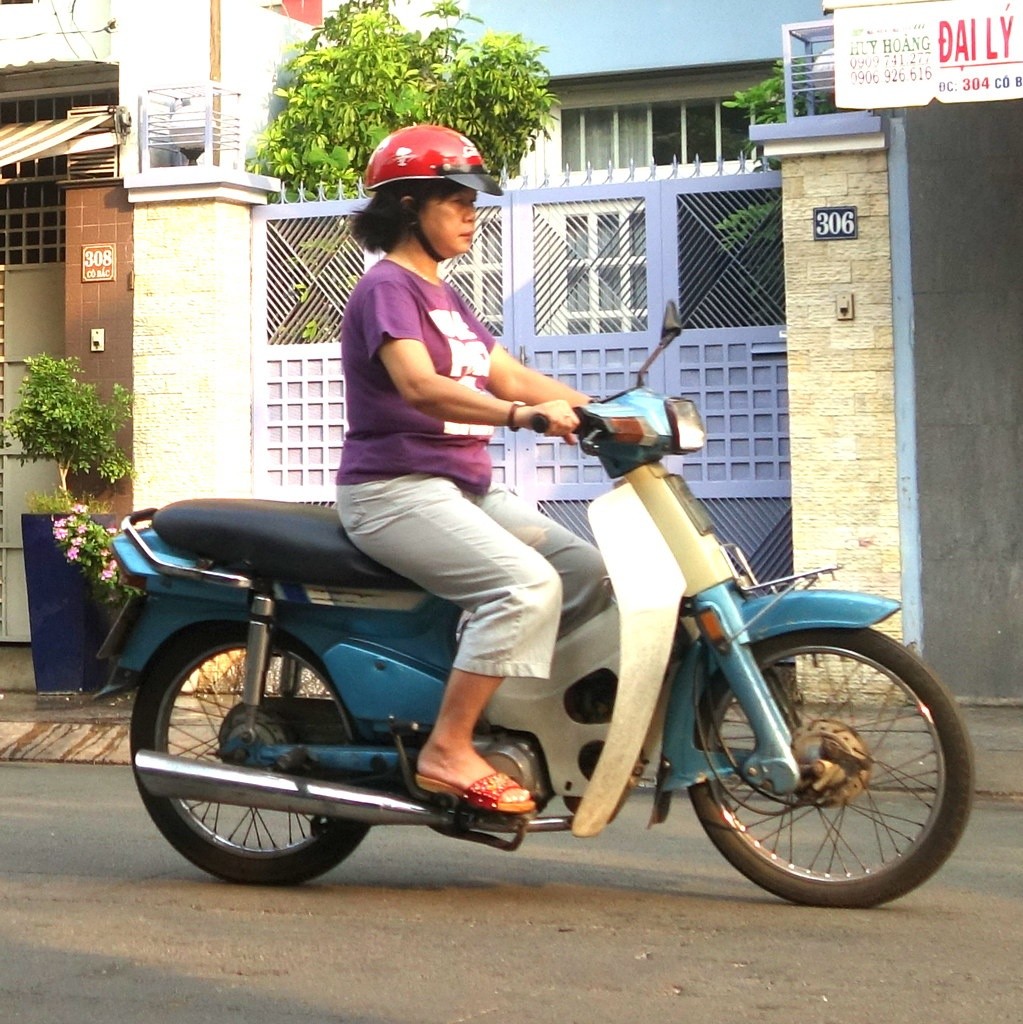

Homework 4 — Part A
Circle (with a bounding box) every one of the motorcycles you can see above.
[85,299,971,908]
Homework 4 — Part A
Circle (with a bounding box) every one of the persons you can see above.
[335,125,598,814]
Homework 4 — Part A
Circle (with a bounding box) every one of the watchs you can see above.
[508,400,526,433]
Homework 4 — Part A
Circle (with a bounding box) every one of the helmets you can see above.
[364,124,504,196]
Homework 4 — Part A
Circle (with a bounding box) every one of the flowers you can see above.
[0,348,146,609]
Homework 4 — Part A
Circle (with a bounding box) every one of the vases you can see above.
[20,509,121,692]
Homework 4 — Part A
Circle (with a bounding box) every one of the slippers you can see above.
[416,772,535,815]
